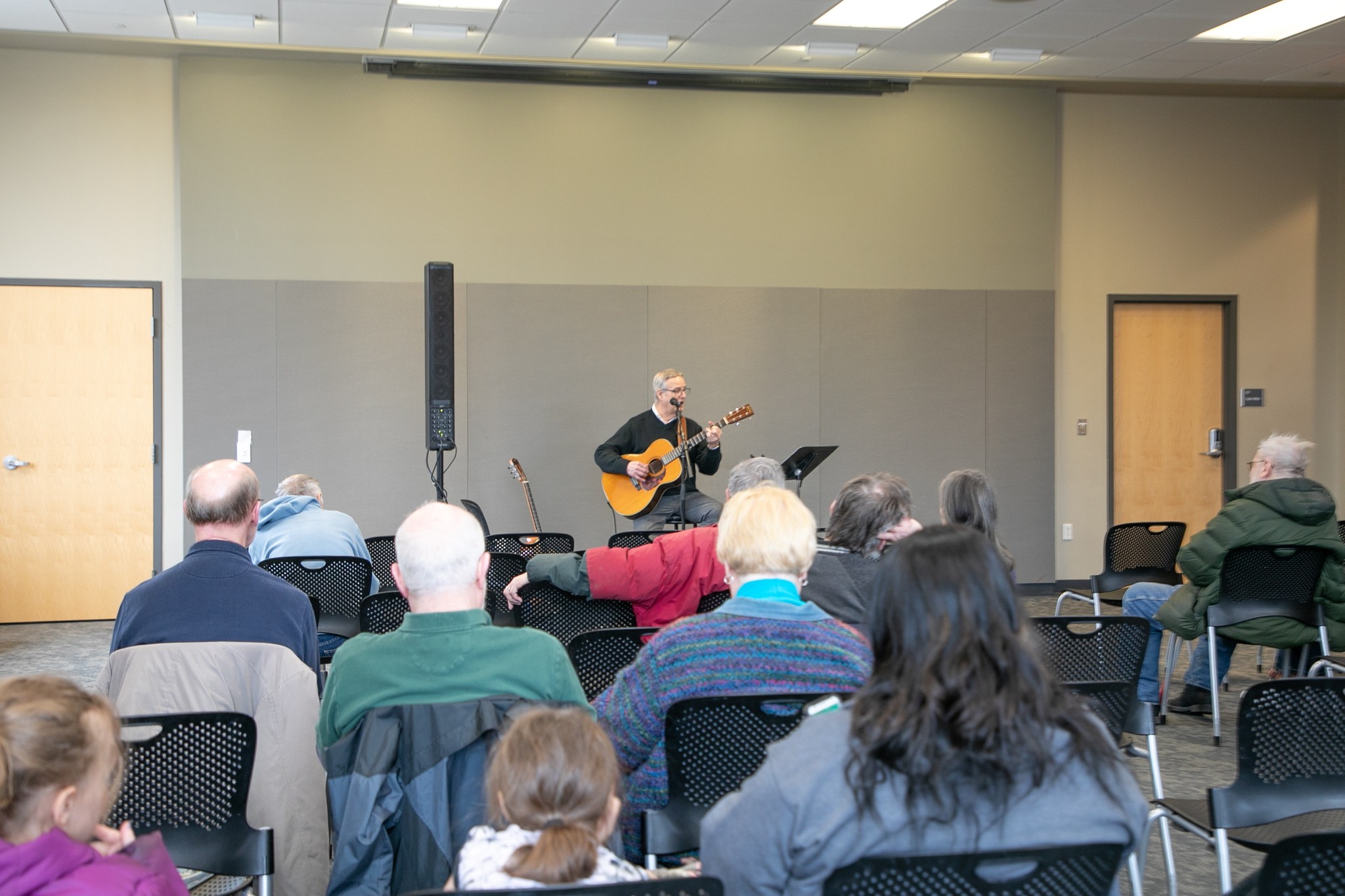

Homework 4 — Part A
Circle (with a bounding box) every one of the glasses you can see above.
[1246,460,1274,472]
[663,388,691,396]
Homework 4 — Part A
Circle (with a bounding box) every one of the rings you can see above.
[711,434,715,438]
[505,586,511,590]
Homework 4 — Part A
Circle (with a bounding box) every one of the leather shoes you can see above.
[1150,703,1160,724]
[1167,684,1212,714]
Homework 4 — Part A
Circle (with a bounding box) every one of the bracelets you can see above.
[707,443,720,450]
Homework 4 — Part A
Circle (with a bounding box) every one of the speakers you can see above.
[423,261,455,449]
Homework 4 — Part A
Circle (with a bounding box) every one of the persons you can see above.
[938,469,1017,584]
[594,368,724,541]
[593,484,870,861]
[698,525,1148,896]
[317,502,602,766]
[248,472,381,660]
[795,473,925,624]
[0,676,192,896]
[503,455,788,645]
[436,708,646,892]
[1122,432,1344,724]
[108,458,319,676]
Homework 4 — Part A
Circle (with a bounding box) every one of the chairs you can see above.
[97,500,1345,896]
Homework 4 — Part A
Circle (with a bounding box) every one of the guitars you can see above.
[601,404,755,520]
[507,457,547,558]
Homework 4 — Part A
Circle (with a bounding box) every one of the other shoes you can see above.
[1118,734,1133,749]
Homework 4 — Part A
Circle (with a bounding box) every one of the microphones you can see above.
[670,398,682,407]
